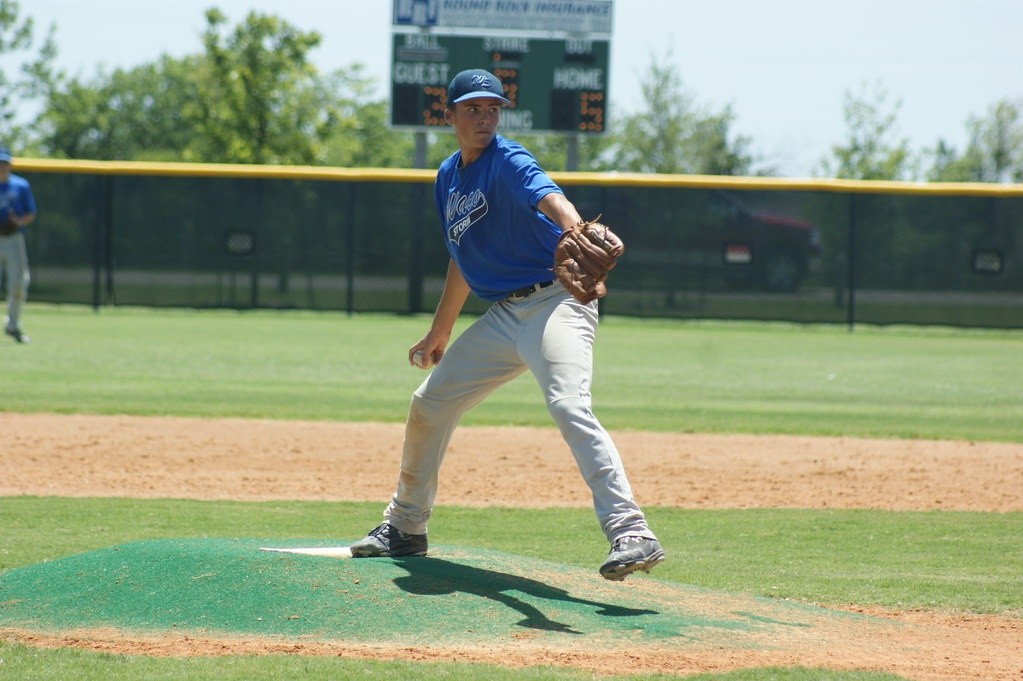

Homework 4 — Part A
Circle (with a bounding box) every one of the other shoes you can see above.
[6,327,28,343]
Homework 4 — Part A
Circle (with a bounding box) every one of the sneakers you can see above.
[599,536,665,582]
[350,523,428,558]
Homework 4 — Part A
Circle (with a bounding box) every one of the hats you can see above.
[447,69,511,105]
[0,148,10,164]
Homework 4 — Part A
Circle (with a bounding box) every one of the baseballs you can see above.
[412,349,434,370]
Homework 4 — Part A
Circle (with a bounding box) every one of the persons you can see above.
[0,148,37,342]
[351,69,664,581]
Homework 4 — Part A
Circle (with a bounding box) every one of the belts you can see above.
[508,280,553,299]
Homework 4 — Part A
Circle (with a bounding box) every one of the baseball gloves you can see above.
[552,221,625,305]
